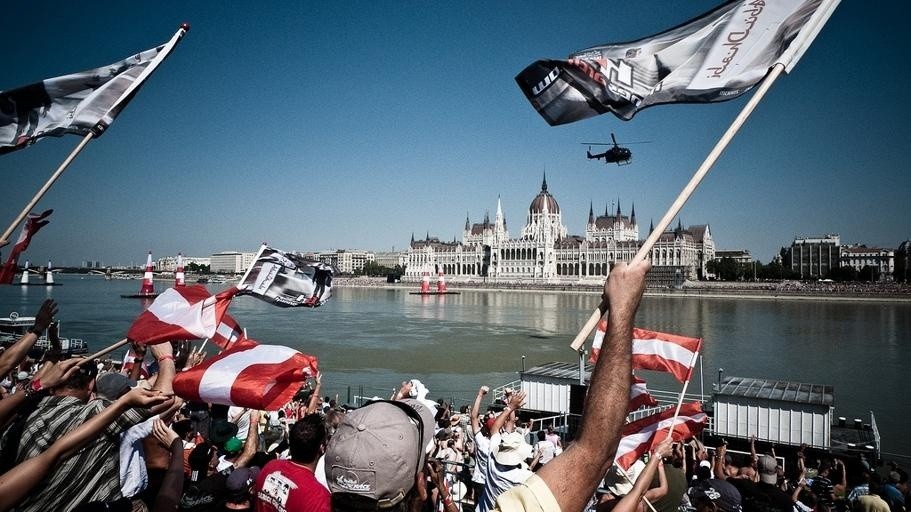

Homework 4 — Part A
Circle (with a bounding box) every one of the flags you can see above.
[0,27,186,156]
[514,0,841,126]
[235,245,334,309]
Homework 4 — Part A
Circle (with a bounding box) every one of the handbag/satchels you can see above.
[554,446,562,456]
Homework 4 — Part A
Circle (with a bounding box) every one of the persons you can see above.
[612,434,911,512]
[0,260,654,512]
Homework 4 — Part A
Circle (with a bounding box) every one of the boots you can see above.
[792,501,814,512]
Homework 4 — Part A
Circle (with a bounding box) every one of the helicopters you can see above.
[575,131,653,168]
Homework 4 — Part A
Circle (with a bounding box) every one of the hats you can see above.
[688,438,777,512]
[451,415,461,425]
[890,471,900,481]
[448,482,468,501]
[96,374,130,400]
[325,398,436,509]
[188,421,260,496]
[18,371,32,380]
[492,433,533,466]
[486,419,496,431]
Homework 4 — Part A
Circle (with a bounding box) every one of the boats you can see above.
[492,341,886,465]
[206,275,226,285]
[0,314,91,361]
[104,268,144,281]
